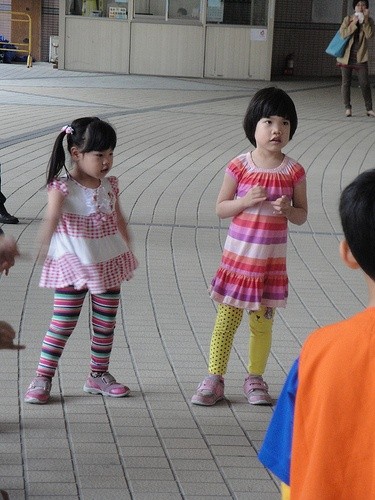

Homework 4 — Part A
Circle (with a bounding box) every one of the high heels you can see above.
[367,110,375,117]
[346,109,351,116]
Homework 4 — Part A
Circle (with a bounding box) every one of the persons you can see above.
[256,168,375,500]
[24,116,139,406]
[190,87,308,407]
[325,0,375,119]
[0,163,28,350]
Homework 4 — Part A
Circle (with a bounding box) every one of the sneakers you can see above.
[242,374,273,405]
[83,370,131,398]
[24,375,52,404]
[0,228,4,236]
[191,375,225,406]
[0,204,19,224]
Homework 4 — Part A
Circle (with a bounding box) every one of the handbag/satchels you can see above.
[325,15,351,57]
